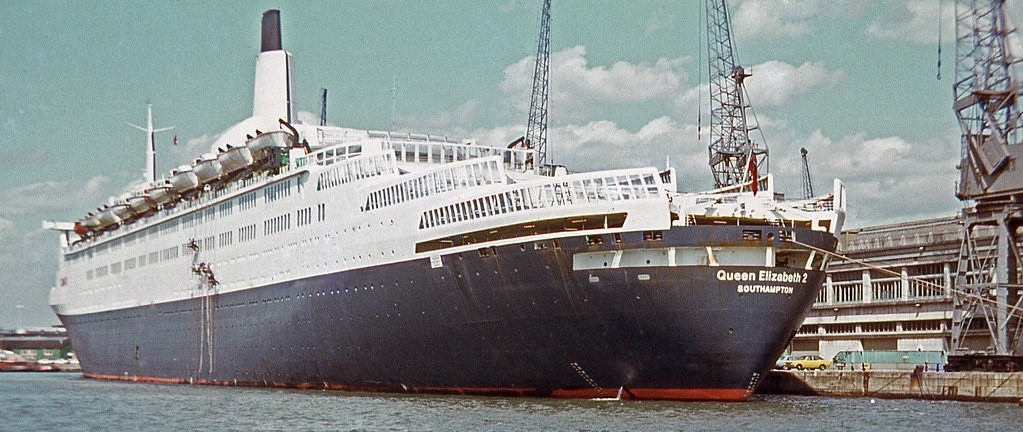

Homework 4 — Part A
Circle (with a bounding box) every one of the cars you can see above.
[785,355,831,371]
[774,355,799,370]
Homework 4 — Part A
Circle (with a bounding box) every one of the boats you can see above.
[0,350,81,371]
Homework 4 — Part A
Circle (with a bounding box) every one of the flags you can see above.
[749,153,759,196]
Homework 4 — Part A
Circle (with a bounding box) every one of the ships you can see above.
[43,9,846,401]
